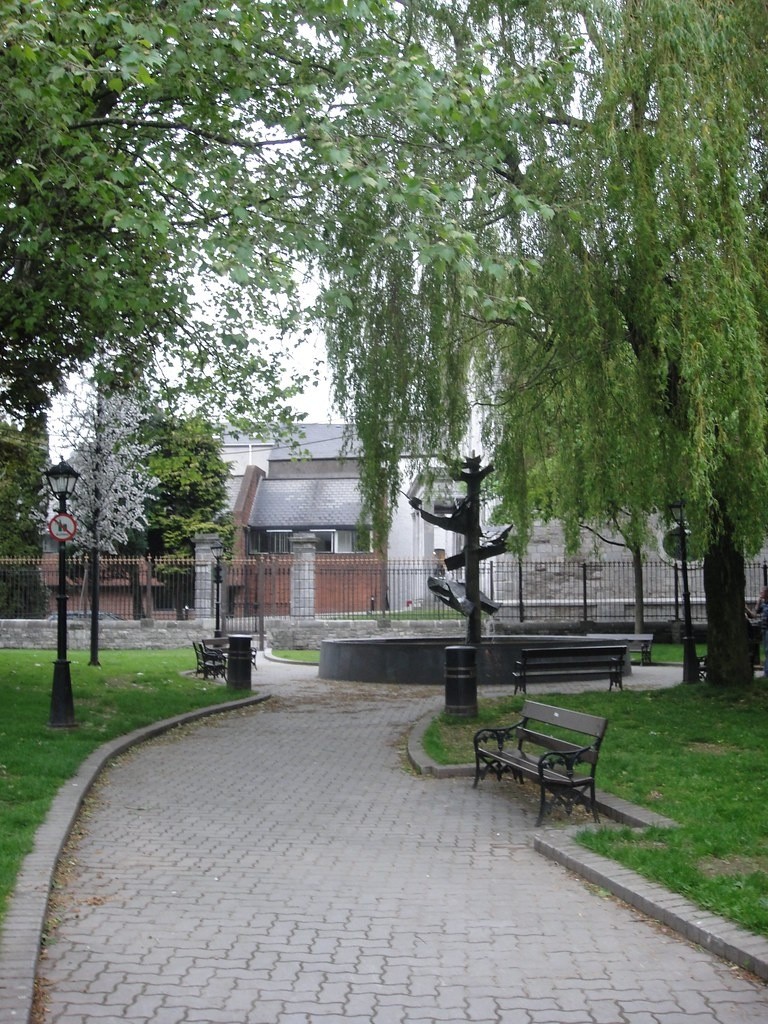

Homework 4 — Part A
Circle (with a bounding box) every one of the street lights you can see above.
[669,493,701,684]
[210,539,225,648]
[45,456,82,727]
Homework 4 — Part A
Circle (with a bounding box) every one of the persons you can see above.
[745,586,768,681]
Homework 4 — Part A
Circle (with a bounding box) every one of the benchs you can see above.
[193,639,257,682]
[511,644,627,695]
[587,634,653,666]
[473,699,608,827]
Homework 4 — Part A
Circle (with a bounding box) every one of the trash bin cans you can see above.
[444,646,479,718]
[227,637,252,690]
[751,620,760,664]
